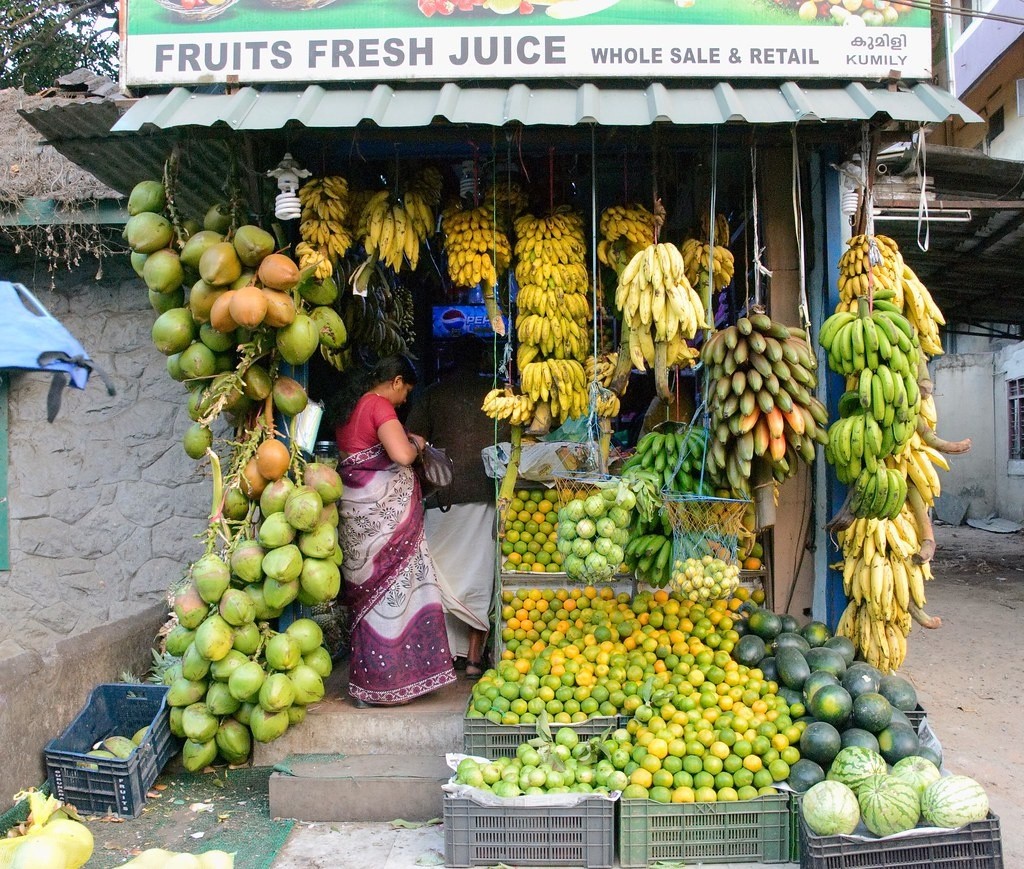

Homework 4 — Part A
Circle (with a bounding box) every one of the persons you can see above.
[405,359,511,678]
[336,356,458,708]
[628,370,680,449]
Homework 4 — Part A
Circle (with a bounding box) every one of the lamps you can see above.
[459,160,476,199]
[267,130,314,220]
[841,174,859,217]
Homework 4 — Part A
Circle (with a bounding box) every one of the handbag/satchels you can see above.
[420,442,455,487]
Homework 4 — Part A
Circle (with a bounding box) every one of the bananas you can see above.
[302,176,971,672]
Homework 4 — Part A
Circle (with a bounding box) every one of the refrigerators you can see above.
[434,305,507,503]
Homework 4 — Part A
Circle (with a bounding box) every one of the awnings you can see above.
[0,282,93,391]
[109,81,985,133]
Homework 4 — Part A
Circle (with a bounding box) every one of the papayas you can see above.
[77,181,349,771]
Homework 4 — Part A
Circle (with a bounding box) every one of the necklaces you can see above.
[371,390,379,396]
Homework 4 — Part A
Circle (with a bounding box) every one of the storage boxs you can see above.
[45,682,180,819]
[441,695,1004,869]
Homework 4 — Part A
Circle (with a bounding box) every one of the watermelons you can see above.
[735,609,989,836]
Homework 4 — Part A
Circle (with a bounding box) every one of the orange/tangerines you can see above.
[456,483,807,802]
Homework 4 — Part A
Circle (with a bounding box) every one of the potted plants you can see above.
[957,484,989,520]
